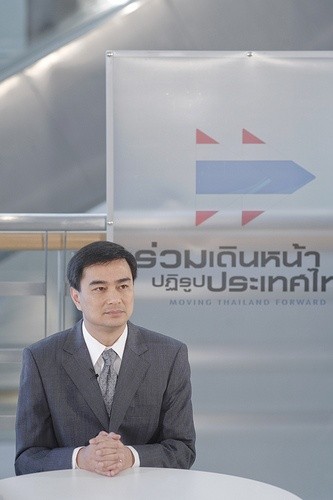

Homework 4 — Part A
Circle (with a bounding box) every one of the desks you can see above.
[0,467,304,500]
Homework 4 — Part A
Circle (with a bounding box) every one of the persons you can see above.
[14,241,196,477]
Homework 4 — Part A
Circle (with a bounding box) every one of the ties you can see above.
[98,348,119,418]
